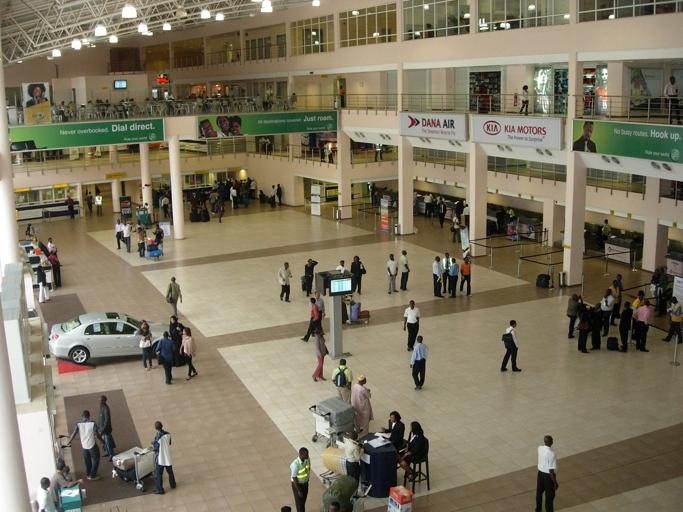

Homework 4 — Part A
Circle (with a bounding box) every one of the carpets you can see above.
[36,293,89,335]
[63,389,160,507]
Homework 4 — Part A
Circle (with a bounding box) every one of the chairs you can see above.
[18,96,257,123]
[378,420,432,493]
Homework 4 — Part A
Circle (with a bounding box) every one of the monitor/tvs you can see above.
[314,270,357,296]
[114,80,127,89]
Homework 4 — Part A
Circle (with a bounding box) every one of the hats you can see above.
[357,374,365,382]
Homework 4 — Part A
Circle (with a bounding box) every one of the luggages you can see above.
[536,272,551,289]
[300,275,309,291]
[315,395,355,441]
[172,340,186,368]
[350,300,362,322]
[112,446,147,482]
[137,241,145,257]
[146,244,163,257]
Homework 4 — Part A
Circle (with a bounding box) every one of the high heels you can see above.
[312,375,327,381]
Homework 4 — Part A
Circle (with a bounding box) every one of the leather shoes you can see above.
[186,372,198,381]
[404,470,418,481]
[661,338,669,342]
[568,333,575,338]
[601,313,620,337]
[636,346,649,352]
[619,345,627,353]
[413,385,422,391]
[145,364,156,370]
[678,341,683,344]
[578,346,600,353]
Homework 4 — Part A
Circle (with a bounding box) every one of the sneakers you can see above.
[279,294,291,303]
[101,453,113,462]
[306,292,312,296]
[499,366,523,372]
[435,290,473,298]
[86,475,102,481]
[388,288,407,294]
[152,489,167,494]
[407,345,415,352]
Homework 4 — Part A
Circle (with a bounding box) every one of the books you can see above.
[367,436,389,449]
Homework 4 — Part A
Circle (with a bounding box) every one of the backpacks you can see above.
[502,332,517,353]
[334,366,348,387]
[606,336,619,351]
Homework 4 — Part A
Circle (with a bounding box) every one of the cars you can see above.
[48,308,169,367]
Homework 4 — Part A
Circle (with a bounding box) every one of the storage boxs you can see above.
[385,484,413,512]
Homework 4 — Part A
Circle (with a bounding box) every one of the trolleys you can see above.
[305,404,359,451]
[345,295,369,325]
[131,449,157,493]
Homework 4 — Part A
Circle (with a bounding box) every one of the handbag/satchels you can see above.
[147,238,153,245]
[360,263,366,275]
[576,320,591,331]
[321,446,358,512]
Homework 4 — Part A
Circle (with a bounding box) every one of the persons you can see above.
[290,446,313,512]
[154,331,176,385]
[396,421,425,481]
[135,206,140,225]
[299,296,323,342]
[263,138,270,154]
[664,76,681,124]
[274,248,472,303]
[339,85,345,106]
[38,476,57,511]
[280,504,290,511]
[342,431,361,499]
[330,357,351,404]
[95,395,115,462]
[381,410,404,450]
[153,175,288,222]
[197,119,216,138]
[61,409,106,480]
[227,115,241,135]
[500,320,522,372]
[408,335,429,389]
[323,141,335,163]
[573,120,596,153]
[534,434,559,511]
[426,23,433,38]
[165,277,182,316]
[25,84,48,106]
[84,192,92,214]
[133,320,153,371]
[167,315,184,366]
[175,323,186,365]
[519,85,529,115]
[114,218,165,259]
[311,329,329,383]
[95,192,102,216]
[258,135,265,153]
[65,195,74,219]
[24,223,35,239]
[54,92,305,120]
[327,501,340,511]
[61,466,72,480]
[36,266,51,304]
[402,300,420,350]
[153,421,176,494]
[602,219,612,238]
[371,180,517,243]
[178,327,198,380]
[144,203,152,224]
[310,290,326,337]
[566,266,683,354]
[351,374,372,432]
[49,456,82,492]
[47,238,62,266]
[47,250,61,287]
[215,115,232,136]
